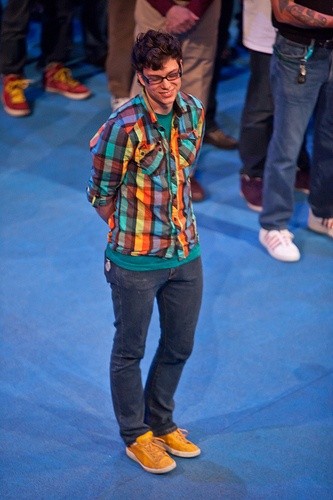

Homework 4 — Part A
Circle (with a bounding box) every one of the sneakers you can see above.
[1,74,32,116]
[43,63,90,99]
[241,174,263,212]
[259,228,301,262]
[308,207,333,237]
[296,167,312,195]
[125,431,177,473]
[153,428,200,457]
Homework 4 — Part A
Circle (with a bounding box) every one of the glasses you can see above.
[140,62,181,84]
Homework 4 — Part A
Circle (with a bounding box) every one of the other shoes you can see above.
[203,129,239,149]
[221,47,238,65]
[111,95,132,113]
[191,177,204,201]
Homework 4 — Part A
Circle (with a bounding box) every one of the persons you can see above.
[106,0,136,110]
[128,0,222,201]
[258,1,333,261]
[235,0,312,212]
[205,0,239,150]
[86,29,204,472]
[0,0,91,115]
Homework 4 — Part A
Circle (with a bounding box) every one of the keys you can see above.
[298,64,307,82]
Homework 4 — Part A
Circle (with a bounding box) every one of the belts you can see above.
[278,29,333,50]
